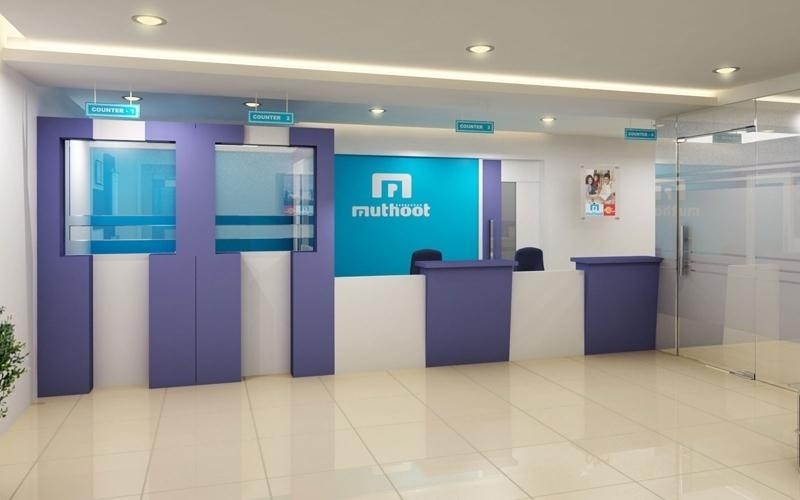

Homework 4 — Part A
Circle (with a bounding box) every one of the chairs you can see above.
[514,248,544,271]
[411,249,441,276]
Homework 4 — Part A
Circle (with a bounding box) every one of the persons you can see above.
[586,173,613,201]
